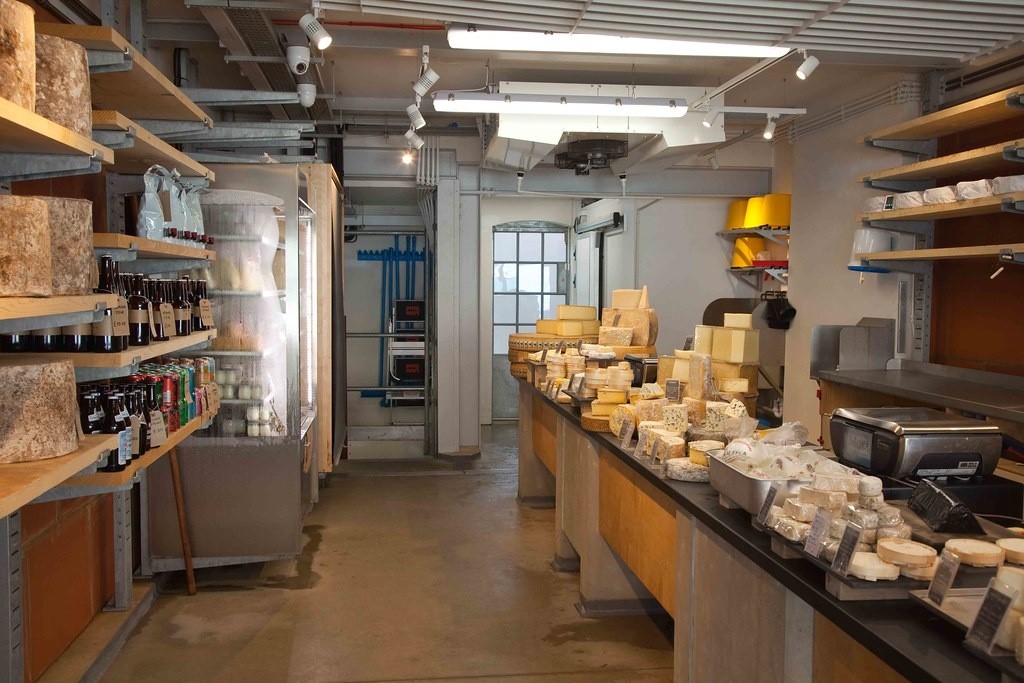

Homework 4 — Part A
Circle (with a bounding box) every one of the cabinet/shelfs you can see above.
[0,96,123,519]
[856,82,1024,273]
[818,378,1023,484]
[0,21,219,489]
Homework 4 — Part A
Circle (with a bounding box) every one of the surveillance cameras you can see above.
[297,84,316,107]
[286,46,311,75]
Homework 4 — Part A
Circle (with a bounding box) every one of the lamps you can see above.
[795,48,820,80]
[430,91,689,118]
[404,122,425,150]
[762,113,780,139]
[411,43,439,98]
[445,24,792,58]
[405,92,426,130]
[298,0,332,51]
[703,98,718,128]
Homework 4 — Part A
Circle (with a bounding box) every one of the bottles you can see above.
[0,253,211,353]
[76,362,196,474]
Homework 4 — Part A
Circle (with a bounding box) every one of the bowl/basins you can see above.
[544,355,584,384]
[722,439,860,482]
